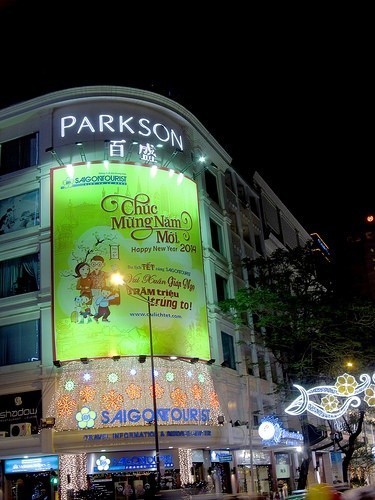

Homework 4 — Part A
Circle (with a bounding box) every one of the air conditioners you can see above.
[10,423,32,437]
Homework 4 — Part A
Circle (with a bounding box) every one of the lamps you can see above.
[46,142,211,186]
